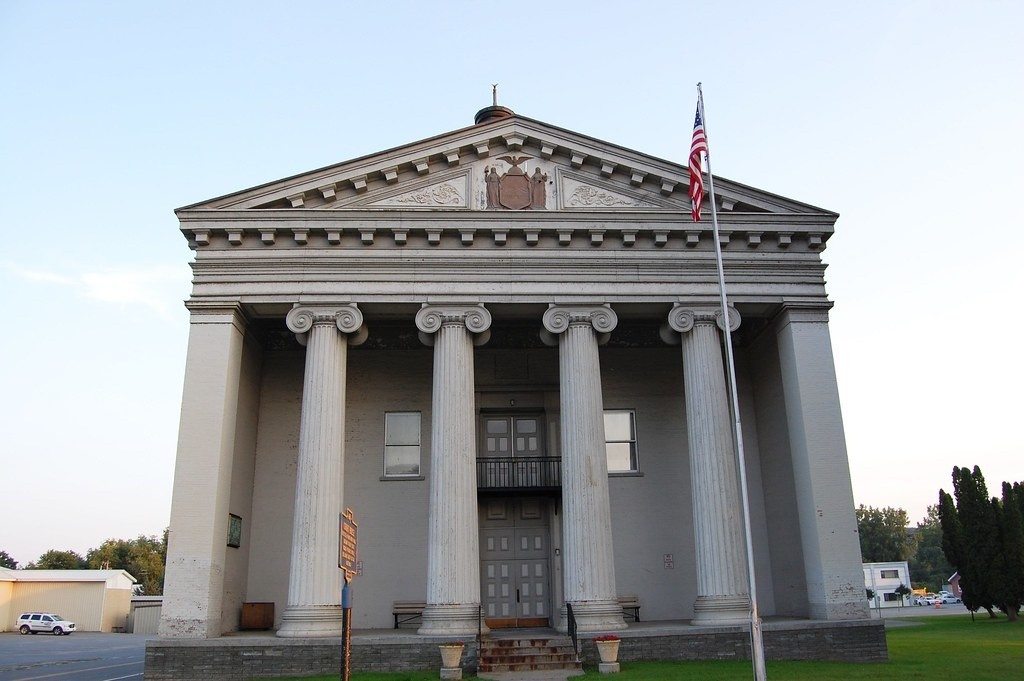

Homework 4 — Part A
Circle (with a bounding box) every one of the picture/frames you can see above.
[227,513,242,547]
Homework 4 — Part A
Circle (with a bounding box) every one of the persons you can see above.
[530,167,547,206]
[484,165,501,208]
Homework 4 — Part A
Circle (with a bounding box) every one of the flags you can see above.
[687,97,708,222]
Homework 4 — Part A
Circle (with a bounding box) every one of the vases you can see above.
[439,646,464,668]
[597,640,621,663]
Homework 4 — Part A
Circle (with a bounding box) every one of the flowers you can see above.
[592,635,619,642]
[444,642,464,646]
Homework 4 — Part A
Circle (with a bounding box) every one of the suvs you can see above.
[16,612,77,636]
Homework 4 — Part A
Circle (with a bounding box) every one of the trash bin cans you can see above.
[239,602,275,628]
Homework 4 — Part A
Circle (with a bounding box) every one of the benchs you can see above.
[392,601,426,629]
[619,595,641,623]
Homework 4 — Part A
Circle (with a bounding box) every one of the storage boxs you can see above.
[112,627,124,633]
[241,603,274,630]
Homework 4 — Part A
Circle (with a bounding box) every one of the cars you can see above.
[911,588,961,606]
